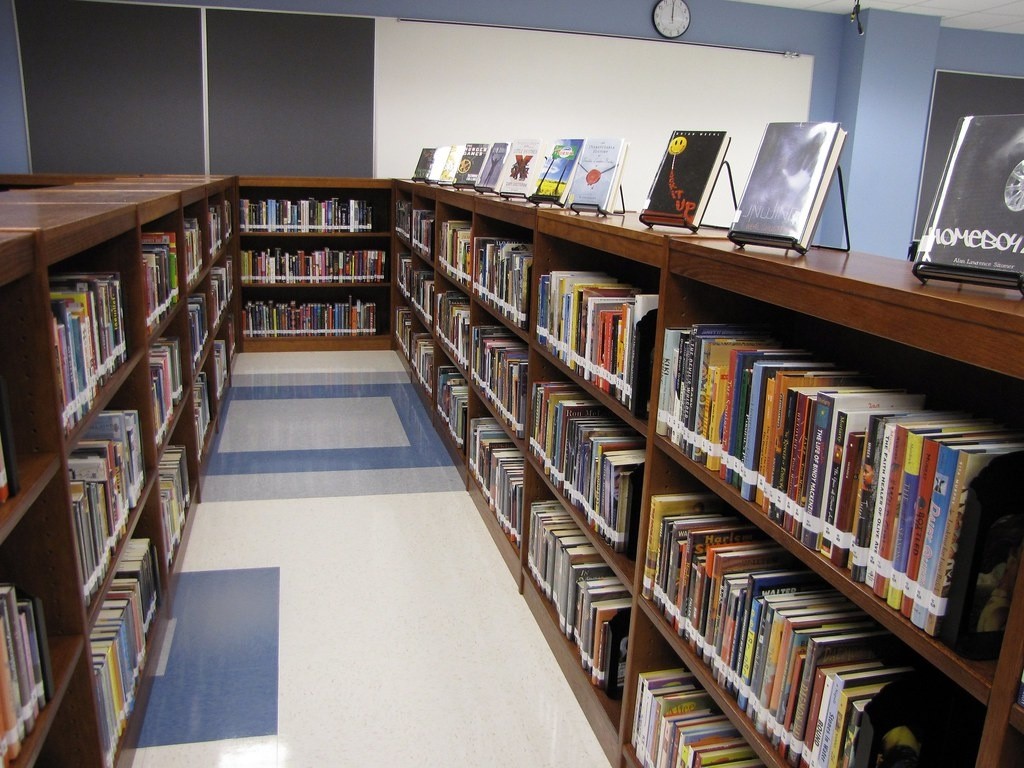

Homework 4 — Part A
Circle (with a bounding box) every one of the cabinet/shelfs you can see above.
[0,171,1024,767]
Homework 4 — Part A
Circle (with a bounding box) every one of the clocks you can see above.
[651,0,692,39]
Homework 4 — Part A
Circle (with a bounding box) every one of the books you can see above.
[411,113,1024,292]
[0,410,20,503]
[242,295,377,338]
[193,372,213,465]
[150,335,184,449]
[214,314,237,401]
[208,200,233,261]
[52,270,131,438]
[186,219,204,292]
[67,409,147,607]
[0,584,56,768]
[141,232,179,336]
[188,293,208,376]
[211,255,235,333]
[239,197,373,233]
[241,248,386,284]
[394,200,660,701]
[157,444,190,574]
[631,321,1023,768]
[91,538,162,768]
